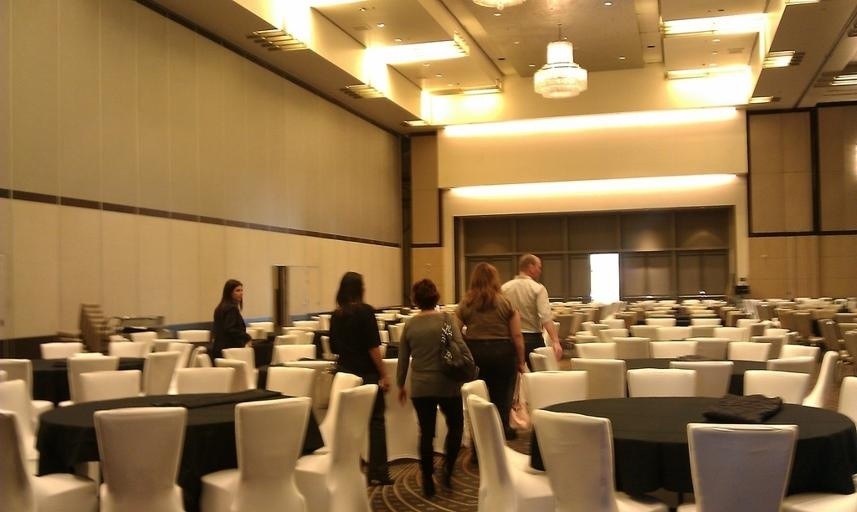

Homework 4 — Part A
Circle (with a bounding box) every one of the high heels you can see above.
[367,471,396,487]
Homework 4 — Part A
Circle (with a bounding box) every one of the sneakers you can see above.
[421,461,454,499]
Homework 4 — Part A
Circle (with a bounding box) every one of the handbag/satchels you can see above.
[439,329,480,382]
[509,370,533,434]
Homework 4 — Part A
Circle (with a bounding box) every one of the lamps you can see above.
[532,22,589,101]
[473,0,527,11]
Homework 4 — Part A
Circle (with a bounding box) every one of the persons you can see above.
[503,252,564,371]
[396,277,475,500]
[327,271,394,487]
[209,279,252,367]
[454,261,527,462]
